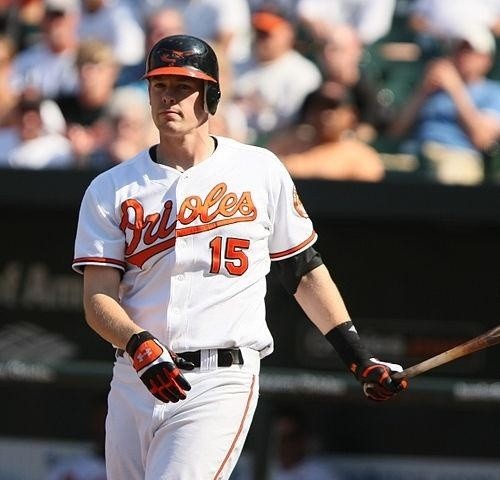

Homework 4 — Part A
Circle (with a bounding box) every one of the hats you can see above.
[0,0,118,107]
[453,26,496,62]
[304,81,350,106]
[248,0,296,32]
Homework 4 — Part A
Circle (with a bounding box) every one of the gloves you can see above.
[324,320,408,401]
[125,330,195,404]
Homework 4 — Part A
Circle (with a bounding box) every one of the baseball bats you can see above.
[392,325,499,380]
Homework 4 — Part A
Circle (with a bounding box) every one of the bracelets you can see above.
[326,320,365,363]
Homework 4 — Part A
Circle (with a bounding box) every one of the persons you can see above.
[0,1,499,183]
[73,34,409,479]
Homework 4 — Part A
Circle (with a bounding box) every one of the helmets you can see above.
[139,34,219,83]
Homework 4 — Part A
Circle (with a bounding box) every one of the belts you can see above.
[115,345,244,368]
